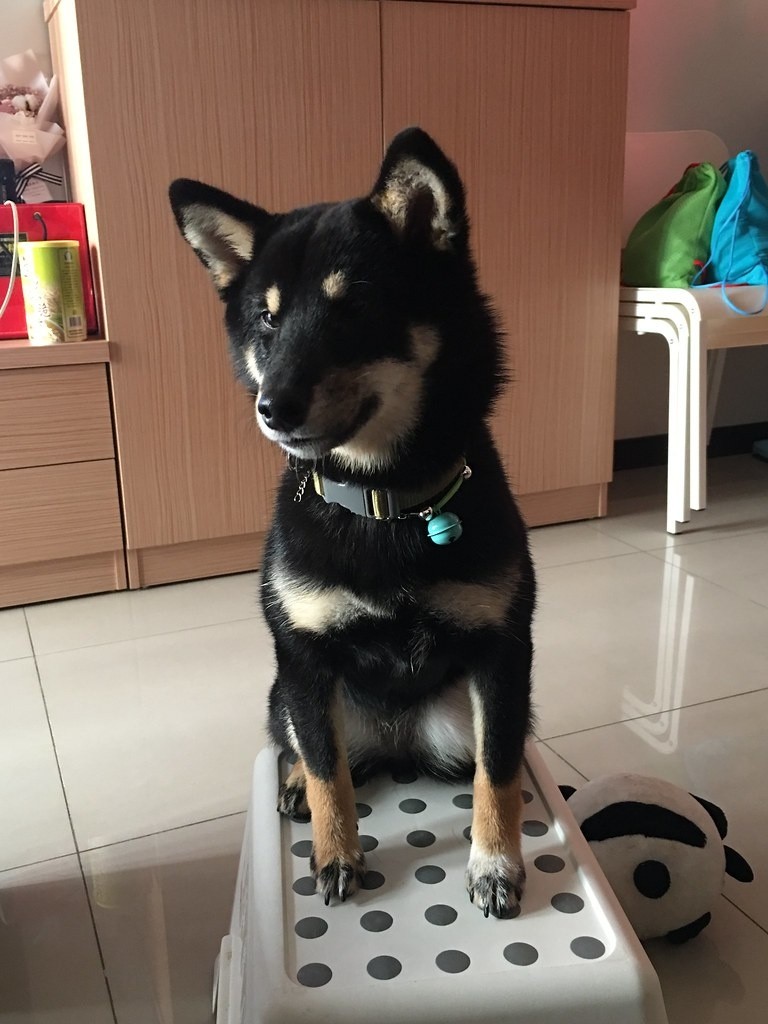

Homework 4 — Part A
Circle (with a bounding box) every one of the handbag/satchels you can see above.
[629,160,728,288]
[688,149,767,316]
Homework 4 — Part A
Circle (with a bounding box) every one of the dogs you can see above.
[168,127,540,918]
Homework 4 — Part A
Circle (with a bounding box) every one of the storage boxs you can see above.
[0,201,98,338]
[211,736,668,1024]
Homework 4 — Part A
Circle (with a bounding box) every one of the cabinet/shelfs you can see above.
[44,0,638,594]
[0,339,128,612]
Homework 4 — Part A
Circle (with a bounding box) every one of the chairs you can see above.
[622,125,768,540]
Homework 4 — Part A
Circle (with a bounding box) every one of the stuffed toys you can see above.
[556,772,754,945]
